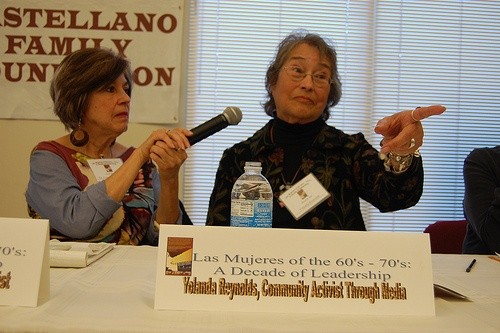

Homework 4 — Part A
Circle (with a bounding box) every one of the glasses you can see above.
[277,66,331,88]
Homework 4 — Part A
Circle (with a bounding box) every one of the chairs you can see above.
[427,219,468,253]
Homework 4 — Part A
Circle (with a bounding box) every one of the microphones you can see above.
[151,106,243,161]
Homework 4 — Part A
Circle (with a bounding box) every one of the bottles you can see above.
[231,162,273,228]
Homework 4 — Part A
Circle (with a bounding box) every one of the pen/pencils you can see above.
[466,259,476,272]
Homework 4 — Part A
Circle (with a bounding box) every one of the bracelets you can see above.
[380,139,420,171]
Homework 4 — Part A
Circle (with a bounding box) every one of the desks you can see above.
[0,244,500,333]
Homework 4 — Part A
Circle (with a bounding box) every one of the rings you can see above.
[175,147,179,151]
[167,130,171,135]
[409,138,415,148]
[411,107,422,121]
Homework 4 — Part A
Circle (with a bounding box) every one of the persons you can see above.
[461,145,500,255]
[25,48,193,246]
[204,34,446,231]
